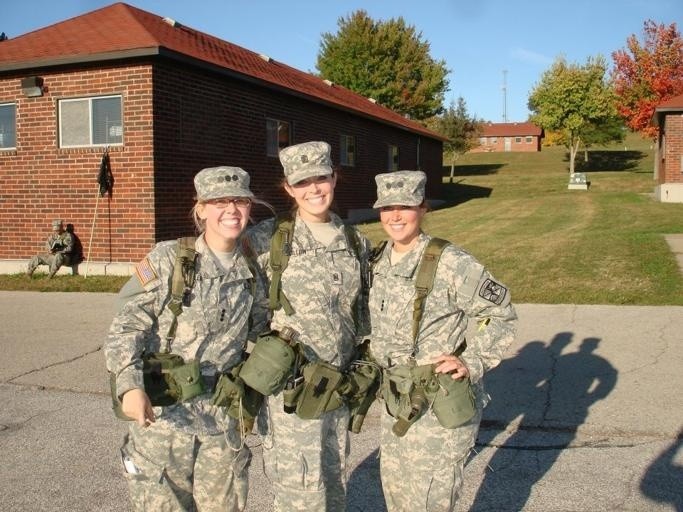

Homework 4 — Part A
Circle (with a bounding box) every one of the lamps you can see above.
[19,75,46,99]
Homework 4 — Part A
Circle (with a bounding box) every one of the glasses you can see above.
[205,199,252,208]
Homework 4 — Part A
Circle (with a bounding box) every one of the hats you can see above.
[52,222,62,230]
[194,165,255,198]
[373,170,426,209]
[279,142,332,185]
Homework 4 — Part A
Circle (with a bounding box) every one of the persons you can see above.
[236,141,376,512]
[25,219,74,282]
[101,164,271,512]
[360,170,518,511]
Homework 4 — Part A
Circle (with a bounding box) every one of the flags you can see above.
[96,152,111,198]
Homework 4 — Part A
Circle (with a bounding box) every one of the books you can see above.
[47,241,60,250]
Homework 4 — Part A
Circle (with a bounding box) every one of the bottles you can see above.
[425,362,477,429]
[239,326,296,396]
